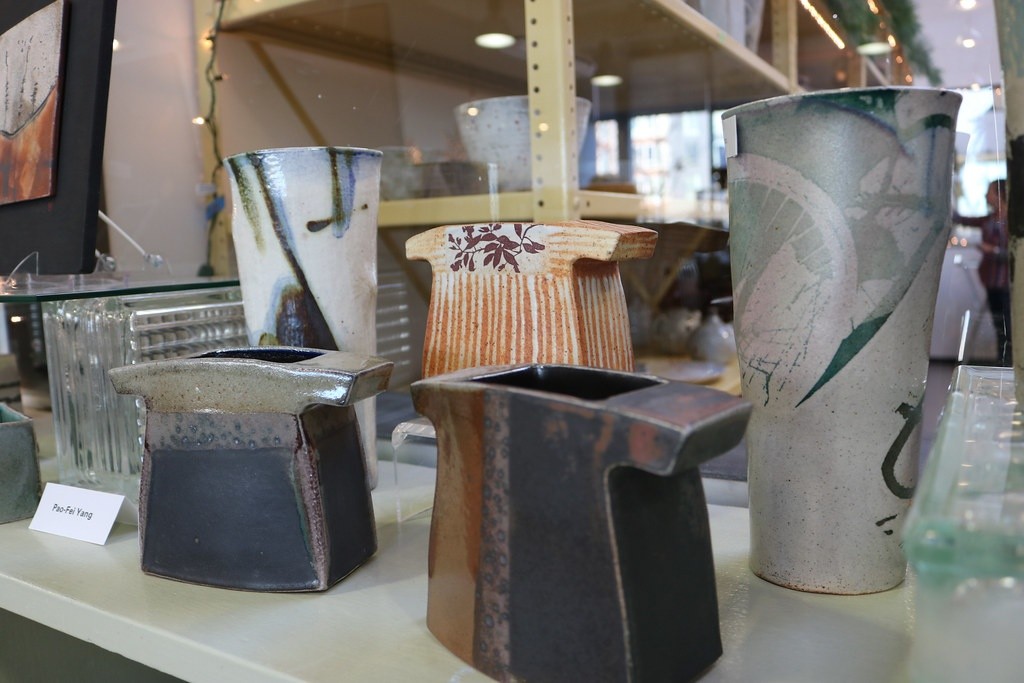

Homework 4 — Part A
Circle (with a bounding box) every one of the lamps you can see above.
[587,38,626,87]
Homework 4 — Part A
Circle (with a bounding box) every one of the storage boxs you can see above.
[0,401,42,525]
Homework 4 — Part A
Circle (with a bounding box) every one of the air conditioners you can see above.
[474,0,517,50]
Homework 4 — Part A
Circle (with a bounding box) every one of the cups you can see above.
[717,82,963,598]
[222,142,383,491]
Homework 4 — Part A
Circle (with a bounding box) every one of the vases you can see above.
[111,345,396,594]
[451,94,593,187]
[406,360,755,683]
[223,144,385,488]
[721,87,965,598]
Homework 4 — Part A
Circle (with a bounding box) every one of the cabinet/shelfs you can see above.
[193,0,898,221]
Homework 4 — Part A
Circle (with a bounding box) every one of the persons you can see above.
[949,180,1012,369]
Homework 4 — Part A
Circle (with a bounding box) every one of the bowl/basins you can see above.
[450,93,594,192]
